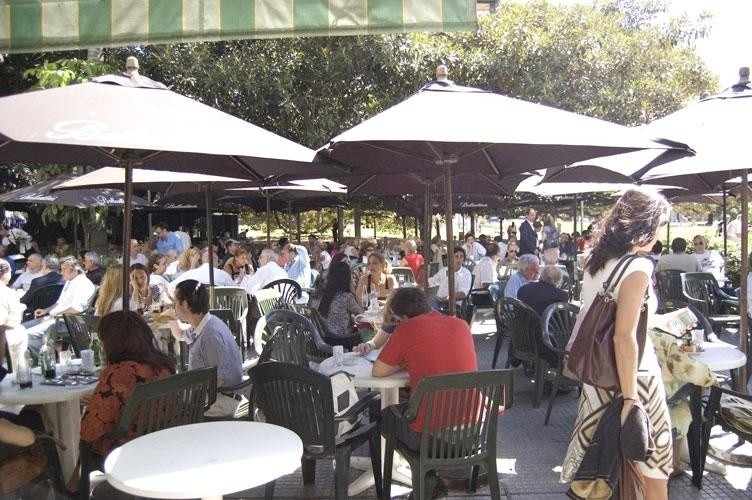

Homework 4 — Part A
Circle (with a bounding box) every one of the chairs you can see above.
[0,429,70,500]
[63,312,113,366]
[456,274,475,324]
[534,303,581,424]
[382,369,517,500]
[390,267,417,288]
[252,290,299,335]
[692,385,752,483]
[487,284,515,370]
[292,303,364,349]
[259,313,347,360]
[655,268,690,313]
[78,365,218,500]
[206,286,249,322]
[205,309,246,361]
[22,285,63,320]
[491,297,584,409]
[463,257,476,269]
[680,272,740,338]
[666,383,702,489]
[417,263,439,289]
[248,359,384,500]
[258,278,303,309]
[350,263,368,280]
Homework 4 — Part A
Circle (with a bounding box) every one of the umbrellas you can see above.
[659,175,752,257]
[1,166,166,257]
[51,164,253,286]
[315,64,698,318]
[534,68,752,445]
[0,55,353,309]
[222,179,348,248]
[516,161,690,259]
[343,173,531,283]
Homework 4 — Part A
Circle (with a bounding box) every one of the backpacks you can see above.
[543,227,557,250]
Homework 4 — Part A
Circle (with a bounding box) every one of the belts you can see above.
[220,389,242,401]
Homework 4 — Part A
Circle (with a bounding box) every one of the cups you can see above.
[42,353,56,381]
[692,329,704,347]
[59,350,71,376]
[81,349,94,374]
[18,359,33,390]
[333,345,343,368]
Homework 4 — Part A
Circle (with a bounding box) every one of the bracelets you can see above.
[365,340,376,350]
[622,396,638,402]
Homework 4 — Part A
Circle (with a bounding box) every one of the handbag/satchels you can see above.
[566,253,656,393]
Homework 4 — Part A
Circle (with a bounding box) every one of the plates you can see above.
[39,371,98,386]
[366,310,382,316]
[343,356,358,366]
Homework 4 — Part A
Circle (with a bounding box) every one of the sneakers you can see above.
[410,470,448,499]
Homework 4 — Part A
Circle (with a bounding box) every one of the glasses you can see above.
[508,250,519,253]
[694,242,706,246]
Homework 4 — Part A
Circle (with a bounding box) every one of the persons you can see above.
[313,261,366,350]
[372,288,480,499]
[494,223,518,272]
[558,188,673,500]
[690,235,736,300]
[95,264,134,318]
[337,235,396,260]
[84,252,106,285]
[12,253,42,290]
[516,266,567,364]
[398,239,423,278]
[474,244,499,291]
[0,214,74,258]
[21,256,96,363]
[149,222,192,257]
[518,209,538,255]
[174,280,245,421]
[655,237,703,275]
[80,311,183,463]
[305,232,333,270]
[19,255,64,321]
[461,231,507,258]
[537,213,557,256]
[351,288,436,356]
[357,252,398,308]
[502,255,539,300]
[427,248,472,313]
[0,258,21,326]
[118,232,311,310]
[559,223,597,258]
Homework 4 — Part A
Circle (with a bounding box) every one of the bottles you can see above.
[41,332,56,379]
[370,282,378,312]
[361,284,369,308]
[90,330,101,367]
